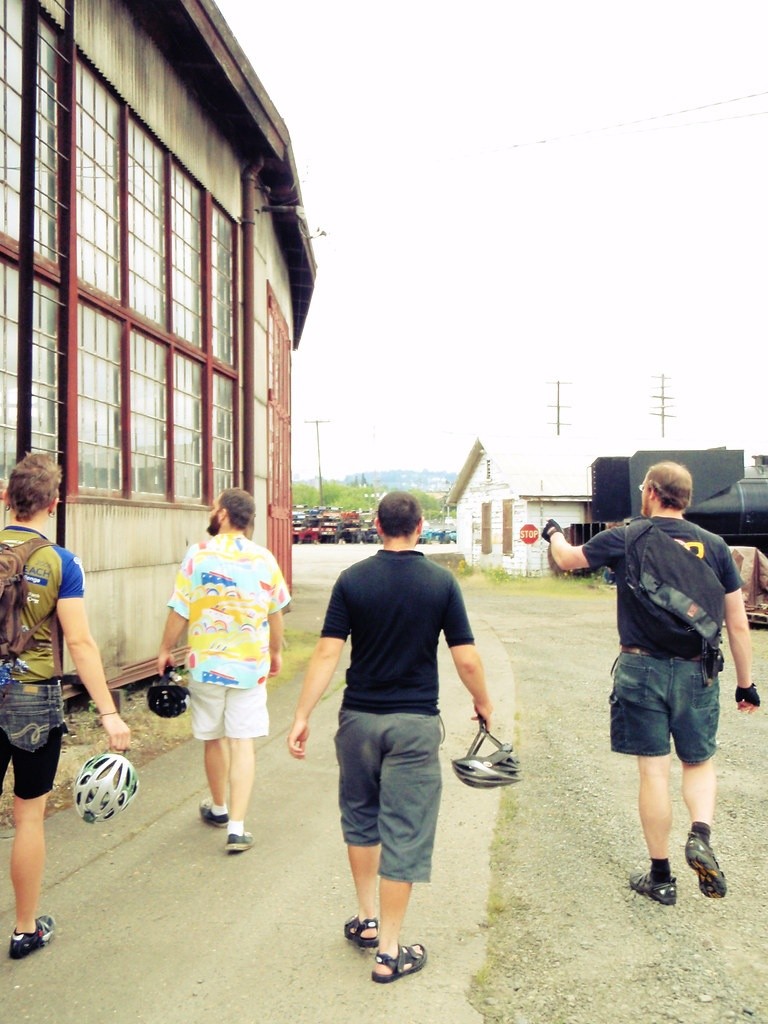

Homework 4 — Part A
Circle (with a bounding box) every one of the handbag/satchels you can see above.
[624,517,724,650]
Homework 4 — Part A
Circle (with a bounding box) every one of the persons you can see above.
[286,489,492,984]
[542,461,760,906]
[1,451,133,960]
[156,487,291,853]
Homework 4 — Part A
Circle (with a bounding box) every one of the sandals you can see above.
[372,943,427,983]
[344,914,379,947]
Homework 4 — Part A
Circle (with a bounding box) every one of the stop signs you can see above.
[520,524,539,544]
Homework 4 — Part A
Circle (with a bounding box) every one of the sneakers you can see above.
[10,915,56,959]
[630,872,678,905]
[200,798,229,828]
[685,832,726,898]
[225,832,254,850]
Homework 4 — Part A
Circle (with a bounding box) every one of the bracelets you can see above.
[99,711,118,718]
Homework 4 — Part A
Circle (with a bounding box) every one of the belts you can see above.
[622,647,703,661]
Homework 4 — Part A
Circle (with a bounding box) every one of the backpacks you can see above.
[0,539,58,661]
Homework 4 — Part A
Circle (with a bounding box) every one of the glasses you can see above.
[639,482,653,491]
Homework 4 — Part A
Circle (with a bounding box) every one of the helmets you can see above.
[72,754,140,824]
[147,686,190,717]
[452,744,522,789]
